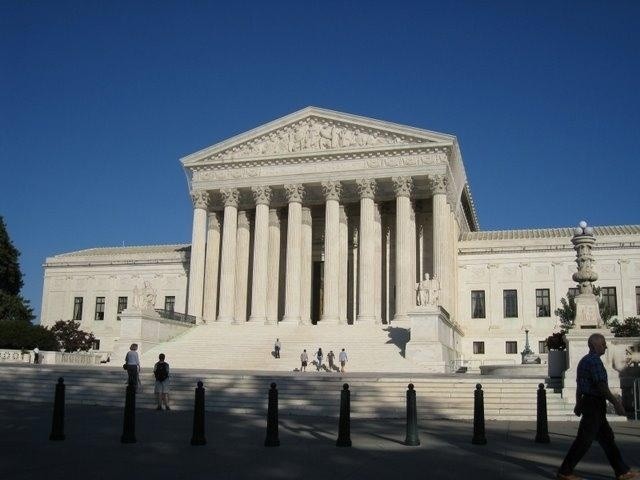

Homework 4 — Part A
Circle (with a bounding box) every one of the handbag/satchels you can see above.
[123,364,127,370]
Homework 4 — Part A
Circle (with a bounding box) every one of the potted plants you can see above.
[545,330,568,379]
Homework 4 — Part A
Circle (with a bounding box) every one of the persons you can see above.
[300,349,308,372]
[338,348,348,373]
[314,347,323,369]
[327,350,336,369]
[417,272,435,307]
[154,353,171,410]
[554,333,640,480]
[33,345,39,363]
[274,337,281,359]
[123,343,140,392]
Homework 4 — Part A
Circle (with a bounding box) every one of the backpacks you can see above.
[154,362,169,382]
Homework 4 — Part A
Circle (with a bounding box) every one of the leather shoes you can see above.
[557,473,582,480]
[616,471,640,480]
